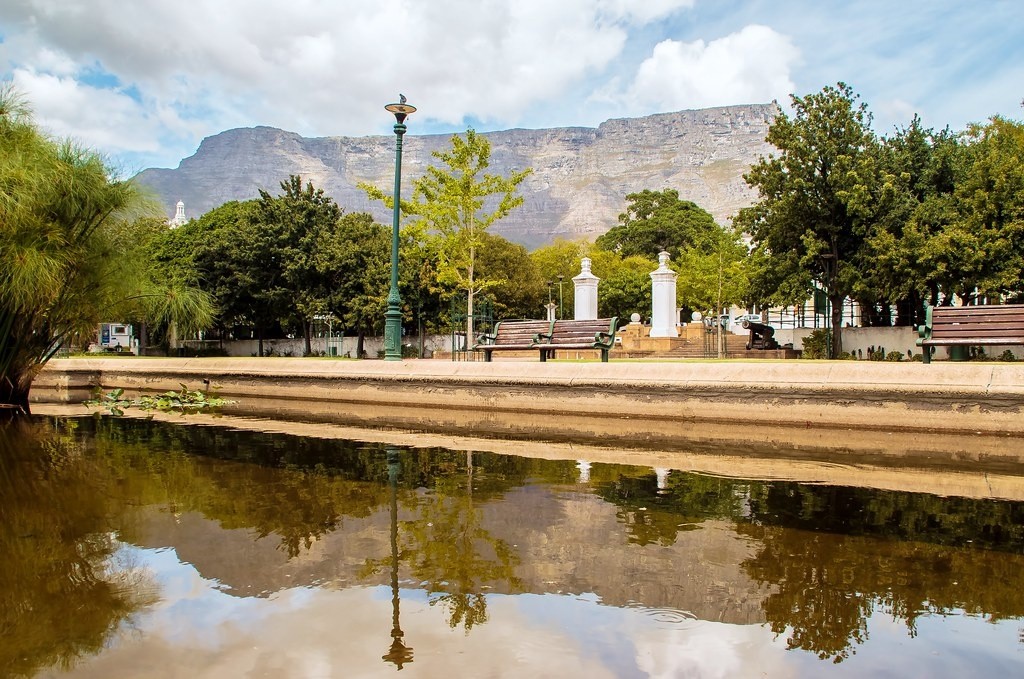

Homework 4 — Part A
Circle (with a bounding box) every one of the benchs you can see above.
[471,313,623,362]
[916,304,1024,364]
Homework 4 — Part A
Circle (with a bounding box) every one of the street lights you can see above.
[383,92,416,361]
[554,273,568,320]
[379,446,415,668]
[544,281,554,322]
